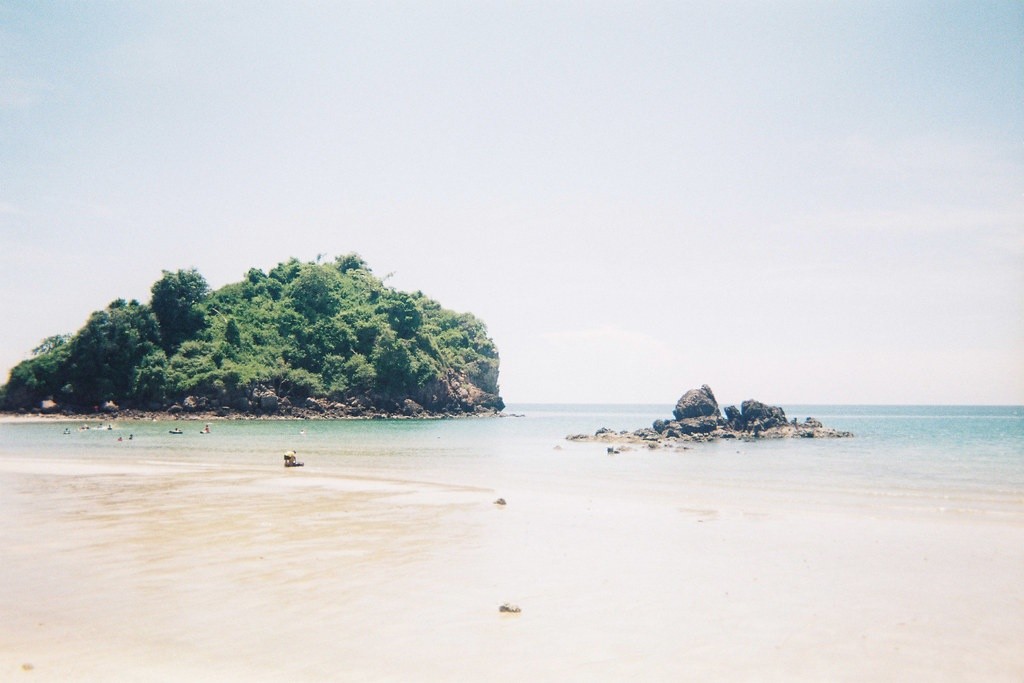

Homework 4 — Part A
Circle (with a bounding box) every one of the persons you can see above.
[284,450,296,465]
[64,421,211,441]
